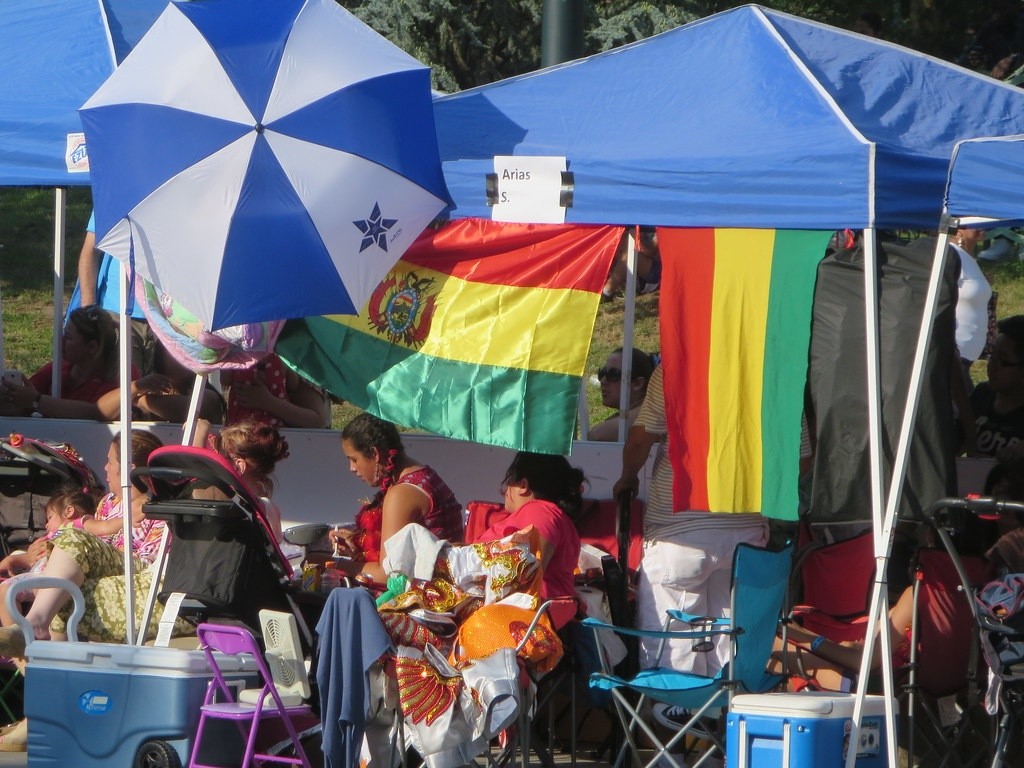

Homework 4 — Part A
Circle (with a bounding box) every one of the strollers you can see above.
[129,443,352,719]
[0,430,107,582]
[928,493,1024,768]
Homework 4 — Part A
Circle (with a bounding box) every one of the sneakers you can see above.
[652,703,716,739]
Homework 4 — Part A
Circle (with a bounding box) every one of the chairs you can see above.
[461,500,618,768]
[188,623,312,768]
[580,536,798,768]
[786,527,989,768]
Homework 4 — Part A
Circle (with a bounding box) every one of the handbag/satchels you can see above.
[63,277,101,330]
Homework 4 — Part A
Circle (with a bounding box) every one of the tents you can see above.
[0,0,170,400]
[430,4,1024,768]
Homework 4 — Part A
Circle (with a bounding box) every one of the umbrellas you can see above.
[78,0,453,334]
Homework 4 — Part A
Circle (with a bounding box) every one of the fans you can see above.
[235,609,310,708]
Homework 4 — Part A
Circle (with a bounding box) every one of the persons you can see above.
[613,365,812,768]
[328,412,463,600]
[474,452,584,692]
[599,227,662,304]
[0,428,196,753]
[0,208,332,429]
[588,348,661,442]
[765,229,1024,717]
[181,419,289,544]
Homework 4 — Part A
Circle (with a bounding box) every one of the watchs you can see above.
[32,392,42,412]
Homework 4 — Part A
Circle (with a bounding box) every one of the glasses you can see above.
[984,352,1024,370]
[596,368,635,384]
[84,304,101,342]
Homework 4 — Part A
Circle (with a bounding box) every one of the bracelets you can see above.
[811,637,826,653]
[337,558,345,567]
[271,397,288,419]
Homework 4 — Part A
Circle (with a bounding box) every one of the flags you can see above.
[273,217,628,457]
[657,226,836,521]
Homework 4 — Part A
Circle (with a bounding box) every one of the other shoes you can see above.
[0,623,38,660]
[977,238,1014,262]
[0,717,27,752]
[654,746,723,768]
[600,288,615,304]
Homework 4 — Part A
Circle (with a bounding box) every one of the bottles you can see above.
[321,562,340,599]
[9,434,40,455]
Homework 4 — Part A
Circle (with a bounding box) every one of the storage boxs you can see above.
[725,690,901,768]
[23,641,261,768]
